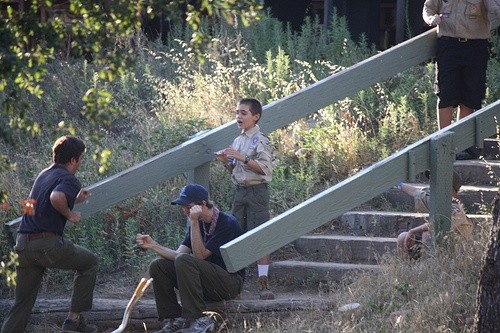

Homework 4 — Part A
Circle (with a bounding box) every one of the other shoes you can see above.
[62,314,99,333]
[183,315,215,333]
[153,318,184,333]
[456,150,473,161]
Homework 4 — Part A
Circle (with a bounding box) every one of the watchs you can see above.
[242,154,250,164]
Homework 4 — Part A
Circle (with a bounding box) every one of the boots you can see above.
[258,275,274,300]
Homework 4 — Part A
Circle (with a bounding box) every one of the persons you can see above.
[136,184,246,333]
[422,0,500,159]
[1,136,99,333]
[397,170,474,264]
[214,98,275,299]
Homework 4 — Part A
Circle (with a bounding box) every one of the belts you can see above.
[441,35,488,43]
[16,231,59,240]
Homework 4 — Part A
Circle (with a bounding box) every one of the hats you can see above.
[425,168,461,193]
[170,184,208,206]
